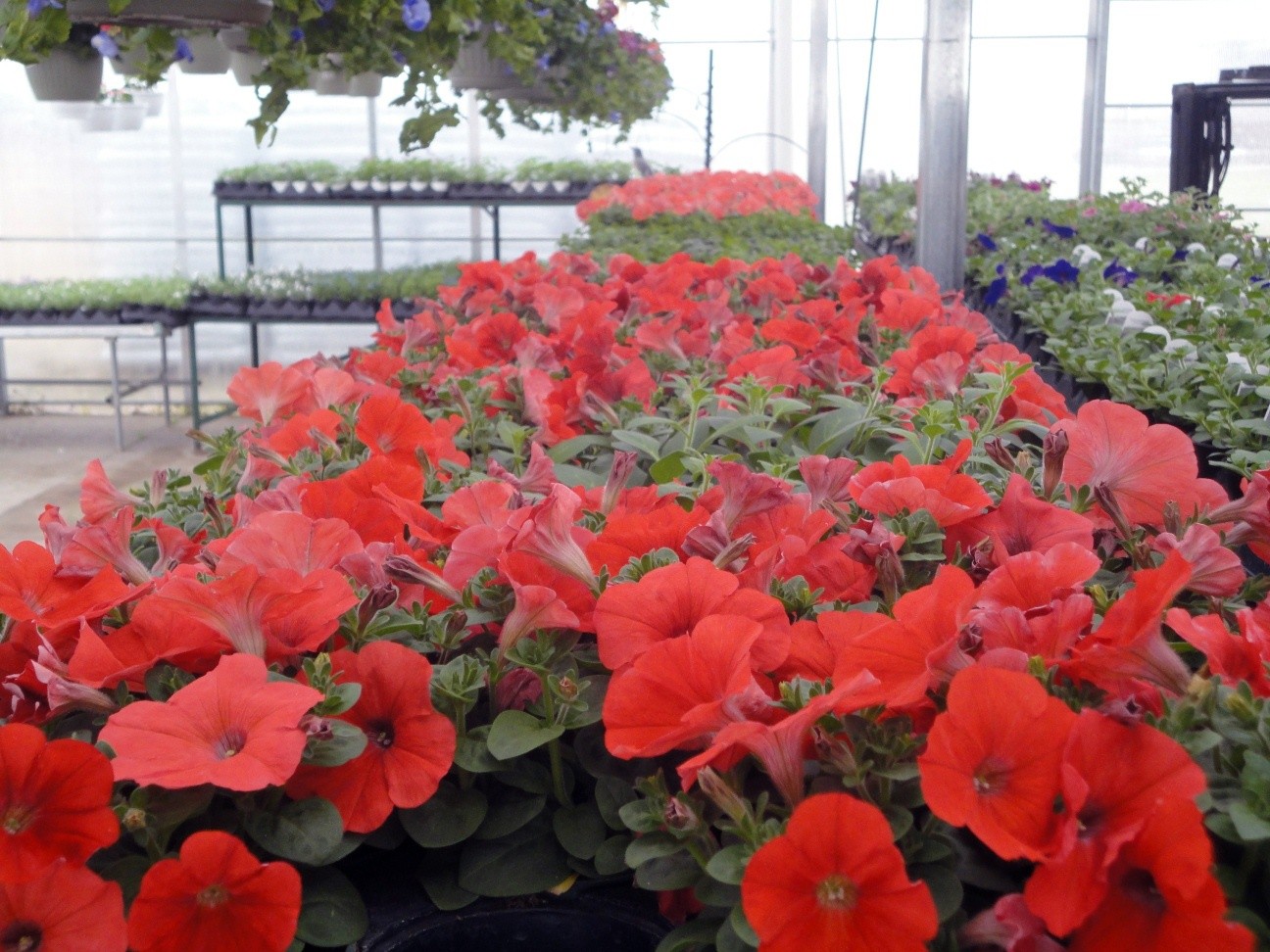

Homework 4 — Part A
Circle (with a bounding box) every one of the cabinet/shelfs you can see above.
[174,191,594,452]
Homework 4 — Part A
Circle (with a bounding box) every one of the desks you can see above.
[0,320,172,452]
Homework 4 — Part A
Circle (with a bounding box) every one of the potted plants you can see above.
[269,156,466,193]
[513,156,573,193]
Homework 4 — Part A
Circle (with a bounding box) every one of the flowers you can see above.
[0,0,672,152]
[0,172,1270,952]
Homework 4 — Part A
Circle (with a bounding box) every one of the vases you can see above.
[230,46,263,86]
[314,52,349,94]
[178,31,232,73]
[22,42,103,101]
[351,69,382,97]
[109,37,147,73]
[449,31,503,87]
